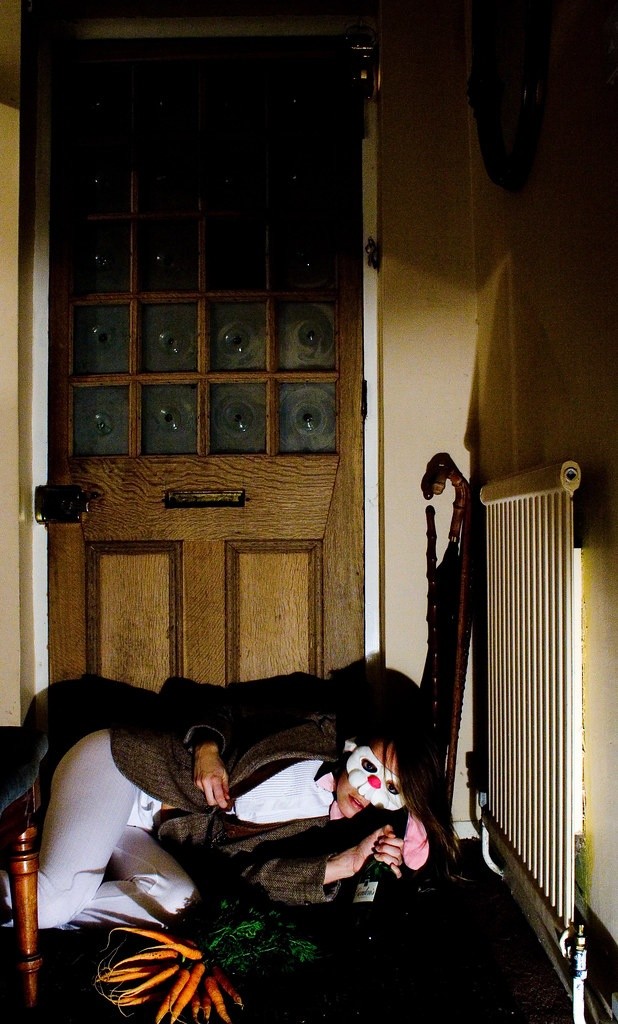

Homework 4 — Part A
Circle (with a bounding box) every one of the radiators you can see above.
[480,460,583,958]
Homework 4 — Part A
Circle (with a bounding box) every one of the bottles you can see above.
[346,857,395,949]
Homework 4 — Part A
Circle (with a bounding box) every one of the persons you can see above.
[0,702,462,931]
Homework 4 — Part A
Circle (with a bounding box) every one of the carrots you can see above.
[94,927,244,1024]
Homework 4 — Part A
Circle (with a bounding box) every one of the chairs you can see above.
[0,726,49,1007]
